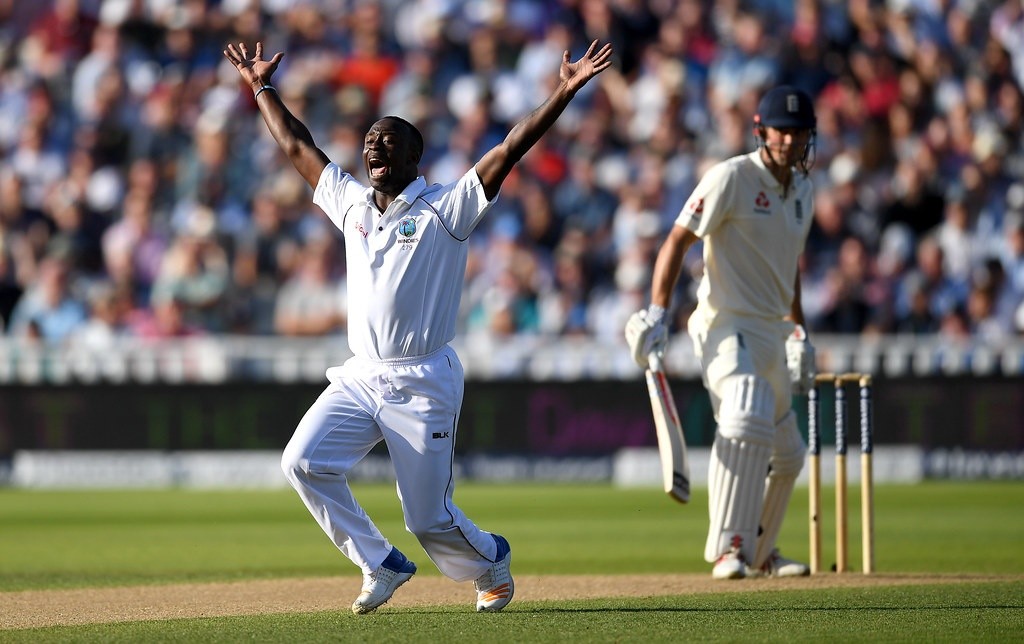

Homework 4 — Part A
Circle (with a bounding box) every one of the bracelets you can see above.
[254,85,277,101]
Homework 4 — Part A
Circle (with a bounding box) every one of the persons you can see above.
[628,82,820,578]
[223,39,615,614]
[0,0,1024,361]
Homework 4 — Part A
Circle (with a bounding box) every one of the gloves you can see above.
[626,304,671,369]
[784,326,817,390]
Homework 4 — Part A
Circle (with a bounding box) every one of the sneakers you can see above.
[472,535,513,612]
[711,552,745,580]
[756,548,809,577]
[352,545,417,613]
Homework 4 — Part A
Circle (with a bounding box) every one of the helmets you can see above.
[753,84,815,128]
